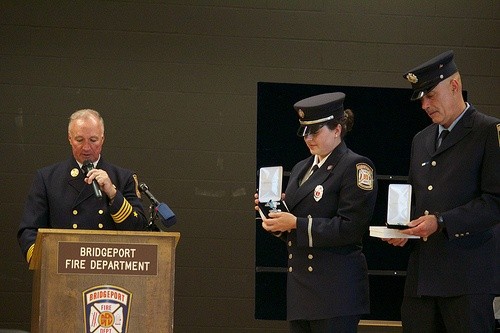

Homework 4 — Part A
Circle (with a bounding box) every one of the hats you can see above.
[294,92,346,137]
[402,50,459,101]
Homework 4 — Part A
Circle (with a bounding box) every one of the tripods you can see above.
[143,205,160,232]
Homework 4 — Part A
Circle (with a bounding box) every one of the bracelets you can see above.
[433,212,444,233]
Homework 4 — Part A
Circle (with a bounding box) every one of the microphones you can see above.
[138,182,177,228]
[83,160,102,200]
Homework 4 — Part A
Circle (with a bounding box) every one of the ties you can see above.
[82,164,88,175]
[441,130,449,144]
[308,164,318,180]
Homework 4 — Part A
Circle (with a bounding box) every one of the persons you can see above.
[16,109,148,264]
[254,91,378,333]
[381,50,500,333]
